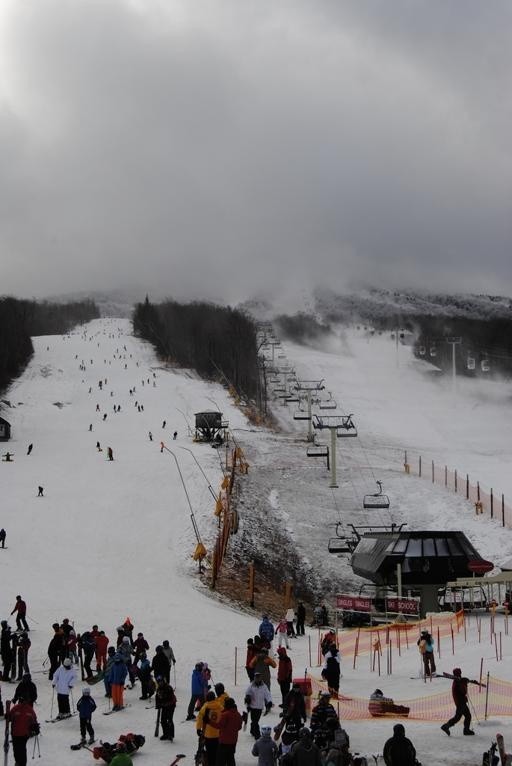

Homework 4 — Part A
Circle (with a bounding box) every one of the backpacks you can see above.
[254,654,270,684]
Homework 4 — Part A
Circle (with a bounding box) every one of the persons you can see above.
[244,601,353,765]
[11,596,30,632]
[47,617,178,766]
[37,486,44,497]
[2,619,41,766]
[85,322,180,462]
[367,689,410,719]
[27,444,33,455]
[0,528,7,549]
[186,662,241,766]
[417,630,435,676]
[440,668,475,736]
[384,724,422,766]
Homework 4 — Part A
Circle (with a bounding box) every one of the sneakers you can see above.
[287,633,305,638]
[139,694,148,700]
[78,739,87,746]
[160,734,174,740]
[186,714,196,720]
[463,728,475,735]
[23,627,30,633]
[2,676,10,681]
[88,738,95,745]
[112,704,122,711]
[46,663,139,699]
[148,691,154,697]
[15,676,22,681]
[16,626,23,633]
[56,712,71,720]
[440,724,450,736]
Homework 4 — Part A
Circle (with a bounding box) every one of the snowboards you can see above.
[86,671,104,685]
[274,699,296,740]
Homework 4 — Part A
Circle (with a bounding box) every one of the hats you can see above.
[293,683,300,689]
[64,658,72,667]
[453,668,461,675]
[123,636,130,643]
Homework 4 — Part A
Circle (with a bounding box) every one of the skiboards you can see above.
[71,740,86,749]
[4,699,10,765]
[103,703,131,715]
[436,672,488,687]
[45,711,79,722]
[483,733,505,766]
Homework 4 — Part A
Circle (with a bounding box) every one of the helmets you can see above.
[261,725,272,738]
[330,627,336,634]
[122,636,130,641]
[319,690,331,700]
[155,674,167,684]
[113,652,122,661]
